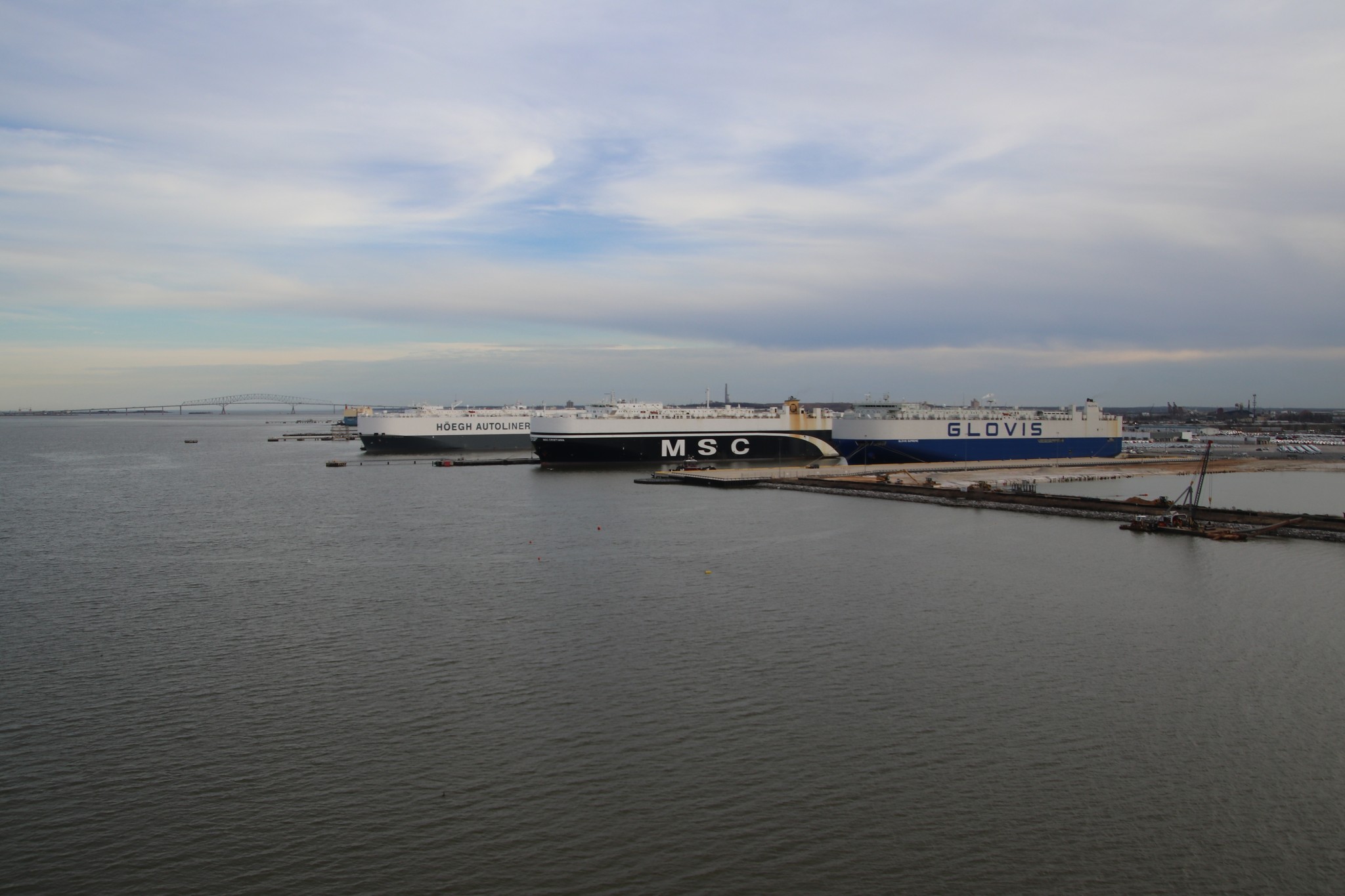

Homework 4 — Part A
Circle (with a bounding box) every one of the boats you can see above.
[354,401,538,453]
[343,404,374,427]
[830,396,1124,466]
[683,454,697,465]
[529,388,837,466]
